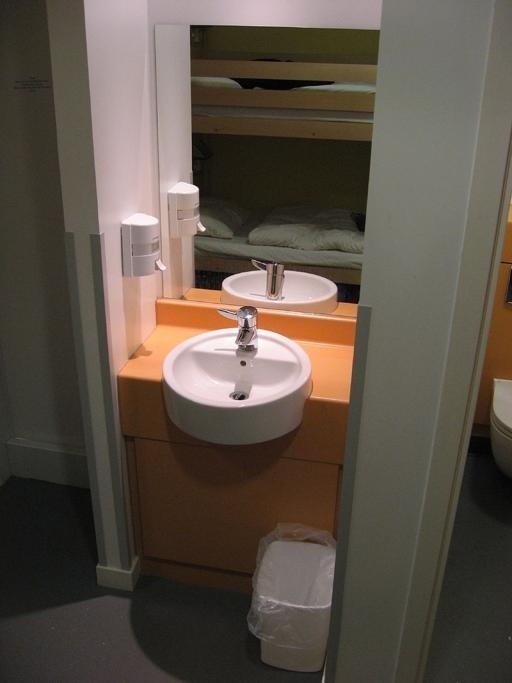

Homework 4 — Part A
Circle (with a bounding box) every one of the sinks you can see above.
[220,269,337,314]
[162,327,311,446]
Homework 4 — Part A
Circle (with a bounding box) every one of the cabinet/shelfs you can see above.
[136,442,340,577]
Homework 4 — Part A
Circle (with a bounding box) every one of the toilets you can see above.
[489,379,512,478]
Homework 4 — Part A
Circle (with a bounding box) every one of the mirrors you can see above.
[154,20,382,347]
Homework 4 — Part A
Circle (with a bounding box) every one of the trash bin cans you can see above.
[254,540,336,673]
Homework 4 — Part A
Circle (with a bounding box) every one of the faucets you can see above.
[250,257,285,298]
[216,307,260,351]
